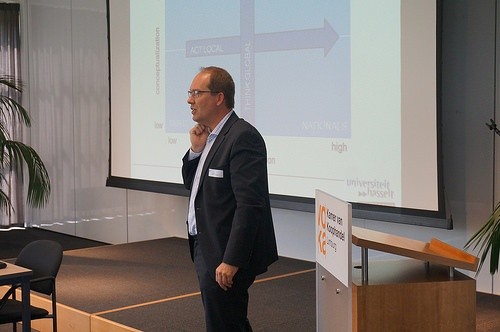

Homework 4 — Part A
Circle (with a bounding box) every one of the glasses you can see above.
[187,90,218,97]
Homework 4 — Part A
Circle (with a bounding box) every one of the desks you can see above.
[0,260,34,332]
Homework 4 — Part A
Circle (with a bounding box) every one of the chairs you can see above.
[0,239,63,332]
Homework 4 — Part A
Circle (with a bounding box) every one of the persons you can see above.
[180,66,282,332]
